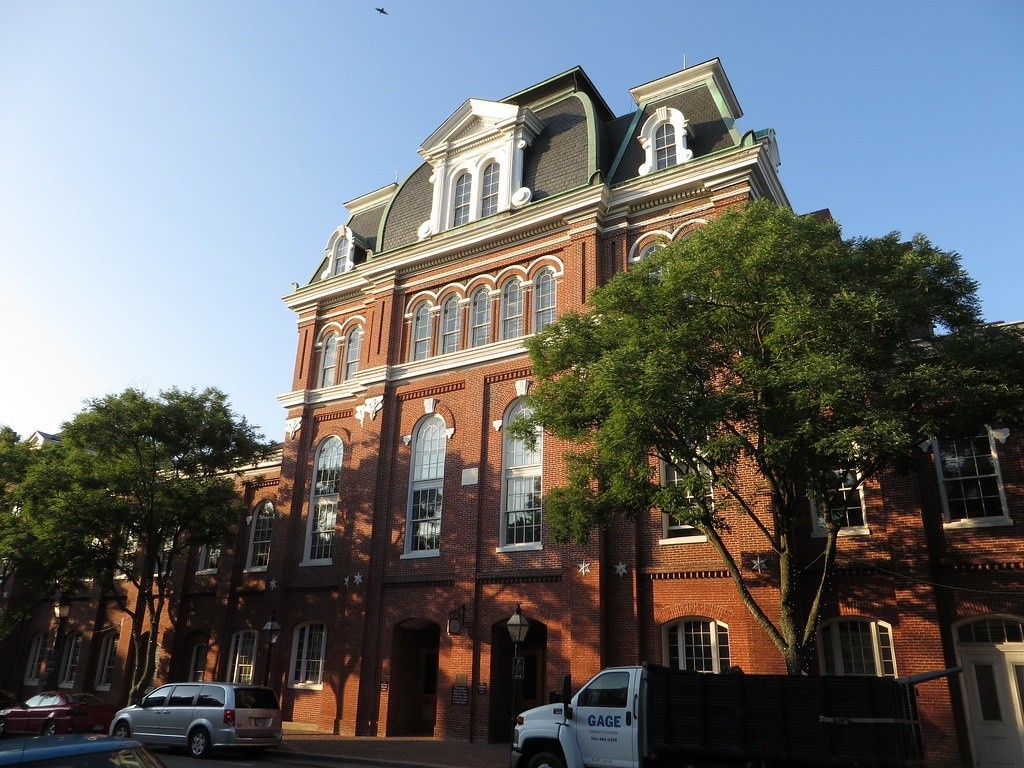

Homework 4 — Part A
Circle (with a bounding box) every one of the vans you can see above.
[109,682,284,755]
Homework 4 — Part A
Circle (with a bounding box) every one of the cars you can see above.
[0,690,116,739]
[20,734,167,768]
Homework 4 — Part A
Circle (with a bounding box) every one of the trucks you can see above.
[512,662,963,768]
